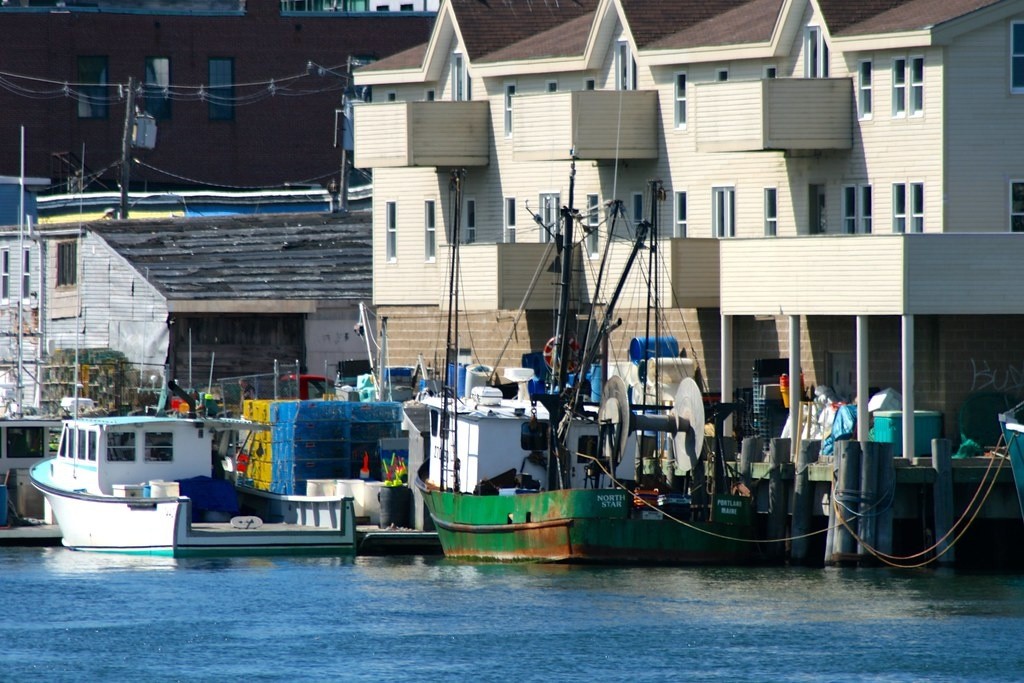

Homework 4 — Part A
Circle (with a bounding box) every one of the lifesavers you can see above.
[543,336,580,374]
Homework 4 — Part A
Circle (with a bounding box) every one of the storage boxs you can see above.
[872,409,941,455]
[732,357,790,452]
[242,359,412,495]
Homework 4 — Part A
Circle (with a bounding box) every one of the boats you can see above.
[414,94,768,567]
[30,142,358,560]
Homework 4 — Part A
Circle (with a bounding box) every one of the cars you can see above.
[280,375,337,403]
[1,420,76,543]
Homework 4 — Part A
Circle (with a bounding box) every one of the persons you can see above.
[190,391,219,442]
[239,379,255,411]
[128,400,145,416]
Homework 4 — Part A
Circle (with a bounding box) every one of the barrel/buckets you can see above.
[590,364,603,402]
[522,349,590,397]
[0,485,8,526]
[306,478,411,530]
[143,480,180,498]
[448,362,495,400]
[626,337,697,450]
